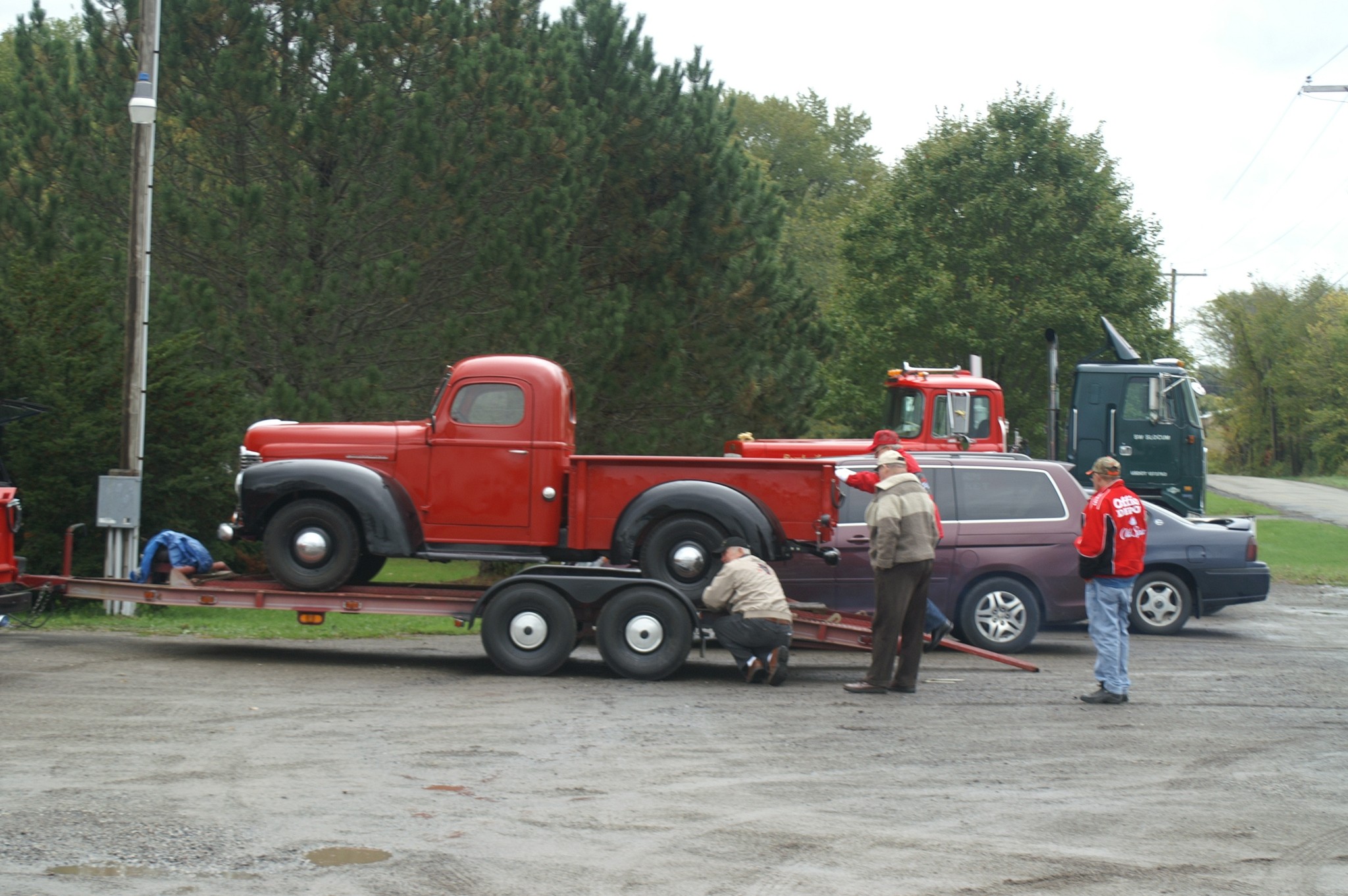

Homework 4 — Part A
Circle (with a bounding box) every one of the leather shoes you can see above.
[926,618,954,651]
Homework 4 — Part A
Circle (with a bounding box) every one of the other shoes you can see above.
[745,657,764,683]
[766,646,789,687]
[843,678,887,694]
[885,681,915,693]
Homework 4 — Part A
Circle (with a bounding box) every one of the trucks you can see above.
[1070,363,1207,517]
[725,366,1009,454]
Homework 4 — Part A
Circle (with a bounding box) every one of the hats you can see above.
[712,536,750,559]
[866,430,900,450]
[873,449,906,471]
[1086,456,1121,475]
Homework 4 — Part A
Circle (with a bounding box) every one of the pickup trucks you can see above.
[218,357,842,600]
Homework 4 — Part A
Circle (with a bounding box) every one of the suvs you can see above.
[757,451,1093,653]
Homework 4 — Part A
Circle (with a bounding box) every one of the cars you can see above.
[1082,489,1273,637]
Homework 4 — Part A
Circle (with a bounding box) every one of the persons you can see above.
[701,536,794,686]
[842,449,941,694]
[1073,456,1148,704]
[833,430,954,651]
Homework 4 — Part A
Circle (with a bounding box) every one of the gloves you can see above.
[834,468,856,483]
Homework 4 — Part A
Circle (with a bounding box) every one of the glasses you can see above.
[873,445,888,456]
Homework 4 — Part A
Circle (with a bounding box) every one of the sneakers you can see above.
[1079,682,1128,703]
